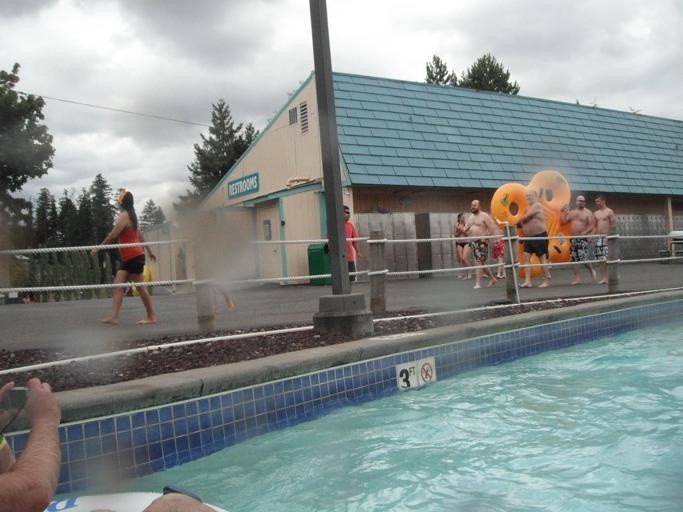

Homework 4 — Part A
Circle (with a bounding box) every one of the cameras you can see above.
[6,387,29,408]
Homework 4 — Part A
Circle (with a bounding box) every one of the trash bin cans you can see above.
[308,243,333,285]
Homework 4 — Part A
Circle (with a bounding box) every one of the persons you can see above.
[591,195,619,285]
[458,198,503,289]
[89,191,163,325]
[123,281,133,296]
[517,190,553,289]
[110,273,115,283]
[20,291,40,304]
[326,204,360,295]
[130,262,153,296]
[0,377,219,512]
[454,213,470,279]
[190,212,242,315]
[560,195,597,285]
[490,239,509,279]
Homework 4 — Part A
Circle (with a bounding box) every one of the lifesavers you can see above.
[130,265,150,298]
